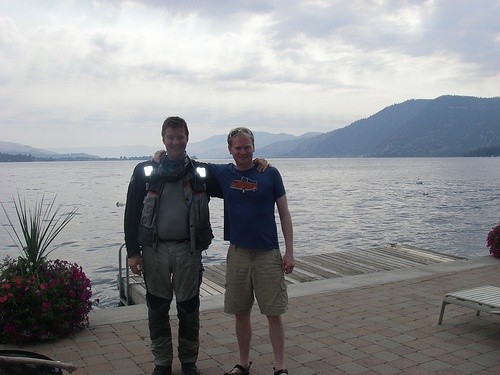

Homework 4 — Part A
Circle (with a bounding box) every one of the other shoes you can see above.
[182,364,200,375]
[151,366,172,375]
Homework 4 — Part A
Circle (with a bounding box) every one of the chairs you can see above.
[437,285,500,328]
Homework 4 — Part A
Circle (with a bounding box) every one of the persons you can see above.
[154,127,296,375]
[124,117,272,375]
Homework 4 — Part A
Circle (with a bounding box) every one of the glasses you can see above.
[228,128,251,141]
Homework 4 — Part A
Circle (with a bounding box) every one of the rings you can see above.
[266,164,268,166]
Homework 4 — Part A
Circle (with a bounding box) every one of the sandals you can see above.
[224,362,252,375]
[273,367,288,375]
